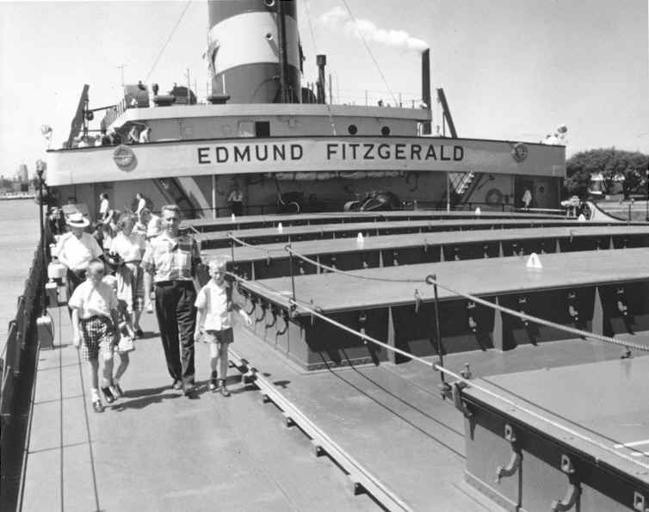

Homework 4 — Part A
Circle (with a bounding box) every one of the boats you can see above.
[1,5,649,510]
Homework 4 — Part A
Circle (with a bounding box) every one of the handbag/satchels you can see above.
[118,337,135,355]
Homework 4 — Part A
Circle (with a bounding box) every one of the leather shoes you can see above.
[128,302,154,341]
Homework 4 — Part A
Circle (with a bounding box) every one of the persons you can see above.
[101,273,136,399]
[42,187,161,341]
[72,123,152,149]
[520,185,533,209]
[139,204,209,397]
[67,259,122,414]
[192,258,253,398]
[224,183,244,217]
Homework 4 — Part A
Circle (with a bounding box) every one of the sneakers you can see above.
[93,378,123,412]
[172,378,230,397]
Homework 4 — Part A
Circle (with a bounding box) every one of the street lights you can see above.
[33,156,48,309]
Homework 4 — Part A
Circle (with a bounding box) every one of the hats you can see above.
[65,213,90,228]
